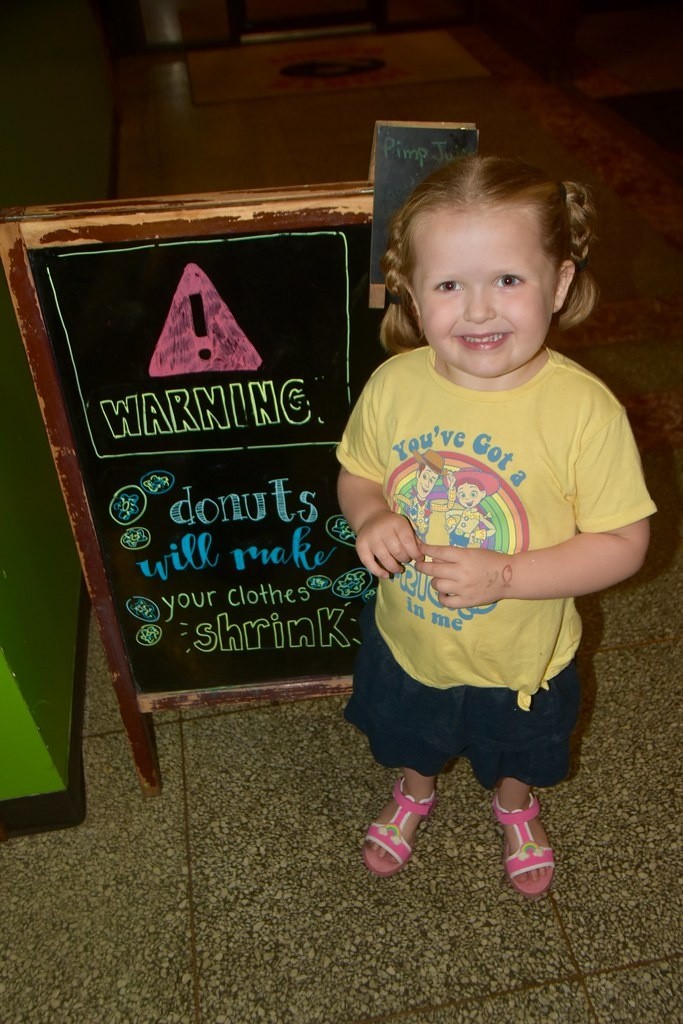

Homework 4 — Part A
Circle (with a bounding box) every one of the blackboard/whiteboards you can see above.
[0,180,380,719]
[369,118,481,288]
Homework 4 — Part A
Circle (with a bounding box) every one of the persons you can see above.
[335,153,659,897]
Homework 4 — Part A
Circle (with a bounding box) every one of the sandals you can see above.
[492,791,555,897]
[363,776,437,877]
